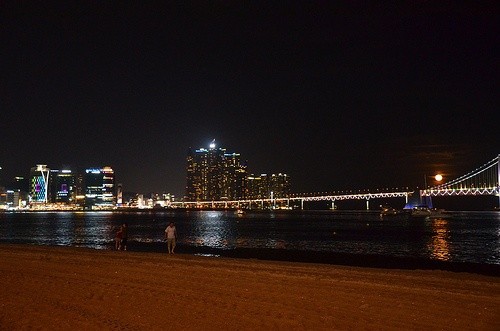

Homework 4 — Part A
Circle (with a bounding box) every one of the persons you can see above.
[114,226,125,251]
[116,223,128,251]
[164,221,178,255]
